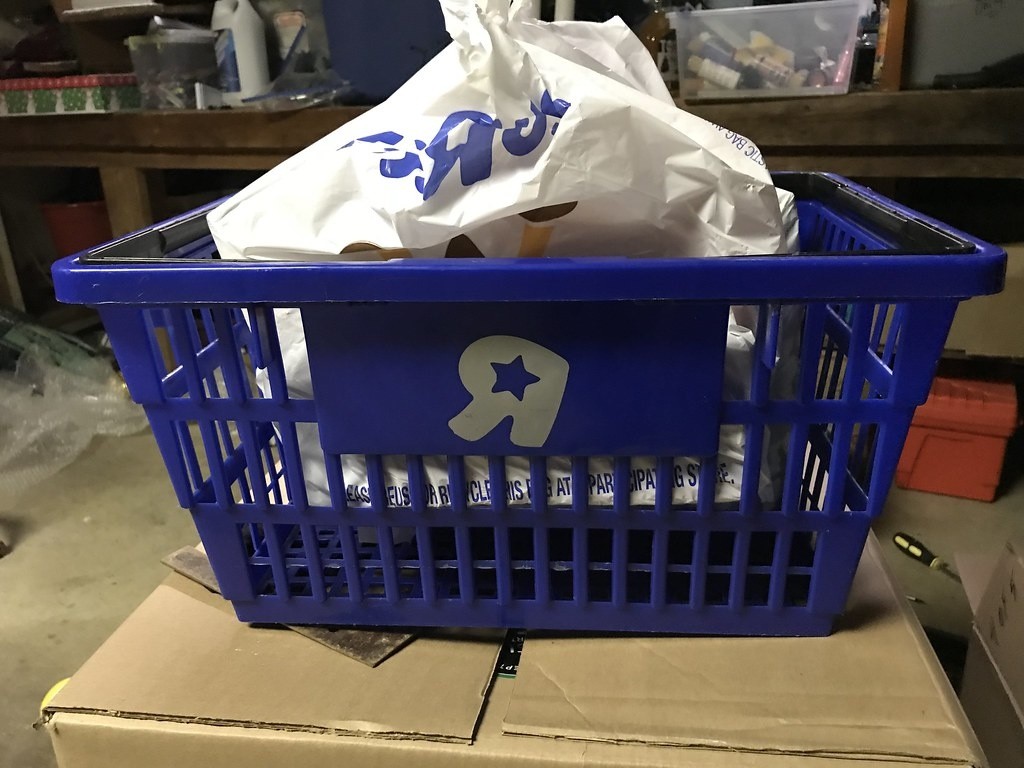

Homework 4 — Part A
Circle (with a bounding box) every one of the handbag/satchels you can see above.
[206,0,805,546]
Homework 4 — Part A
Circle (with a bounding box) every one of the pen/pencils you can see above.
[907,596,926,604]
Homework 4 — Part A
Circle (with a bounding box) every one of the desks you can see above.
[0,85,1024,381]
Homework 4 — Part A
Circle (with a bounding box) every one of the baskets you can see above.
[51,167,1010,639]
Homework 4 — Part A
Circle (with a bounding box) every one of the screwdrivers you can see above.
[891,532,961,584]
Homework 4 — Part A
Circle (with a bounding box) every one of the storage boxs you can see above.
[664,0,859,100]
[38,517,991,768]
[50,169,1007,638]
[322,0,454,107]
[955,539,1024,768]
[893,375,1017,502]
[858,244,1024,359]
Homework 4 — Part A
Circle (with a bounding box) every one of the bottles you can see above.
[210,0,270,108]
